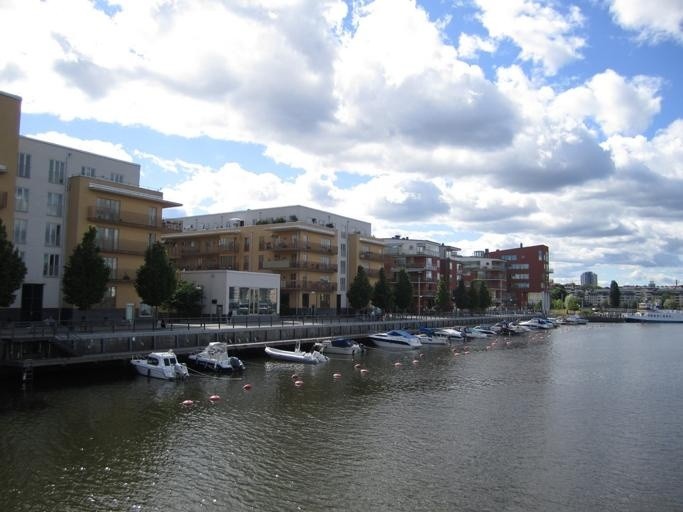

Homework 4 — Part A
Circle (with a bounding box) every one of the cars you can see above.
[361,307,385,320]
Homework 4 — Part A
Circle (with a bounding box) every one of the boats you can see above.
[313,339,364,354]
[621,302,683,322]
[367,315,587,348]
[130,341,246,378]
[264,347,329,362]
